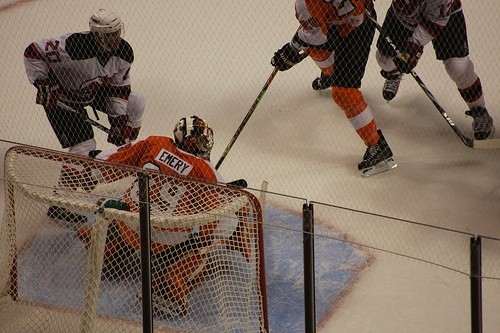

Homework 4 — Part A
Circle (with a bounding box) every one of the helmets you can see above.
[173,116,214,162]
[89,9,122,33]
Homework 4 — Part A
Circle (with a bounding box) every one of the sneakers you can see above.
[465,106,495,141]
[358,130,397,177]
[381,69,403,102]
[46,191,88,226]
[312,71,338,95]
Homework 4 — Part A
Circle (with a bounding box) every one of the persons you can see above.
[376,0,497,140]
[24,9,147,231]
[270,0,397,177]
[82,116,256,318]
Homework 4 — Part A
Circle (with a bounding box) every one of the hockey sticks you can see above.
[367,13,500,148]
[225,178,247,189]
[57,101,130,143]
[215,68,278,171]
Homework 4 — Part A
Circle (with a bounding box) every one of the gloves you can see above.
[393,41,423,74]
[271,32,310,71]
[32,79,57,111]
[107,114,132,147]
